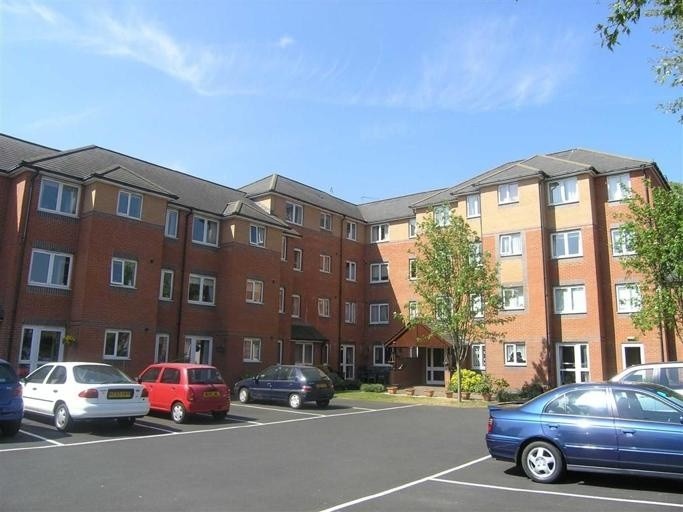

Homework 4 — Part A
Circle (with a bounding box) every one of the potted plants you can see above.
[480,384,492,401]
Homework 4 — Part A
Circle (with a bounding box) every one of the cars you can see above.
[230,362,336,410]
[0,358,28,440]
[481,380,682,487]
[17,360,152,433]
[131,362,233,425]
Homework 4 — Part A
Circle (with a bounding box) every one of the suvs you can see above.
[573,359,682,413]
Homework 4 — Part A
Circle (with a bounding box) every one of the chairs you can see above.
[660,374,669,386]
[617,397,641,418]
